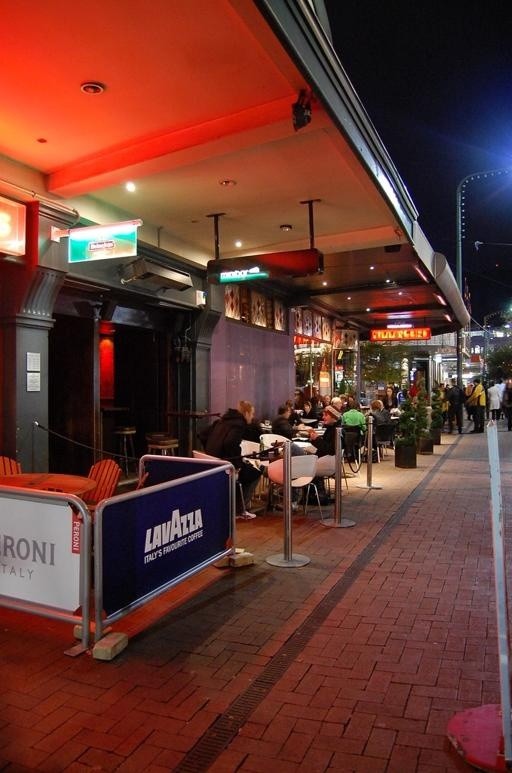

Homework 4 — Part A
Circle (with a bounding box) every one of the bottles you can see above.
[260,436,264,451]
[274,439,278,455]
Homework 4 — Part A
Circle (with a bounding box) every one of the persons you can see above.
[196,400,262,521]
[437,377,512,434]
[267,387,410,513]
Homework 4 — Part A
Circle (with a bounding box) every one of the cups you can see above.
[319,402,323,407]
[318,422,322,428]
[265,420,269,425]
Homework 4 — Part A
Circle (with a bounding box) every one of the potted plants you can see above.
[394,368,448,469]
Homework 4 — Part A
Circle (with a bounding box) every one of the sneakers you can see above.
[235,510,257,521]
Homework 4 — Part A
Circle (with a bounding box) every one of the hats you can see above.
[323,404,342,421]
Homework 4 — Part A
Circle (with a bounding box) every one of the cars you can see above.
[360,391,368,405]
